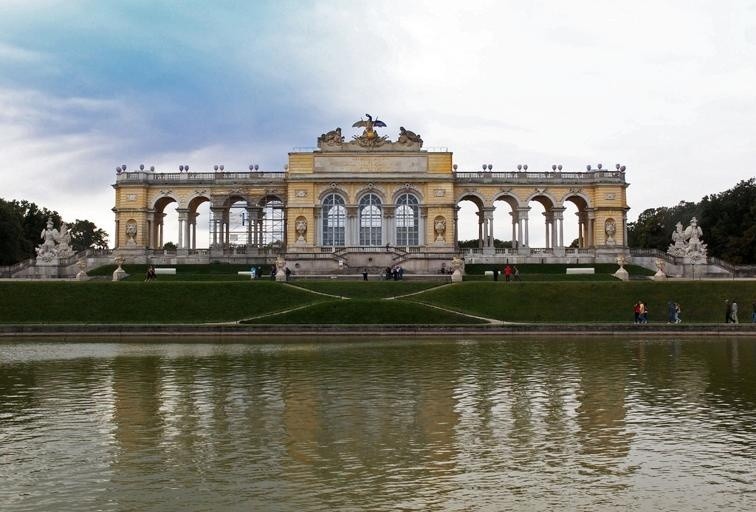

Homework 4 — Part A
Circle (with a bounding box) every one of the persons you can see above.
[512,265,522,281]
[144,264,157,283]
[730,298,739,324]
[492,267,500,281]
[361,268,368,281]
[751,299,756,323]
[383,265,403,281]
[632,299,649,325]
[674,303,683,324]
[724,299,736,323]
[503,266,513,281]
[250,264,291,282]
[668,301,677,324]
